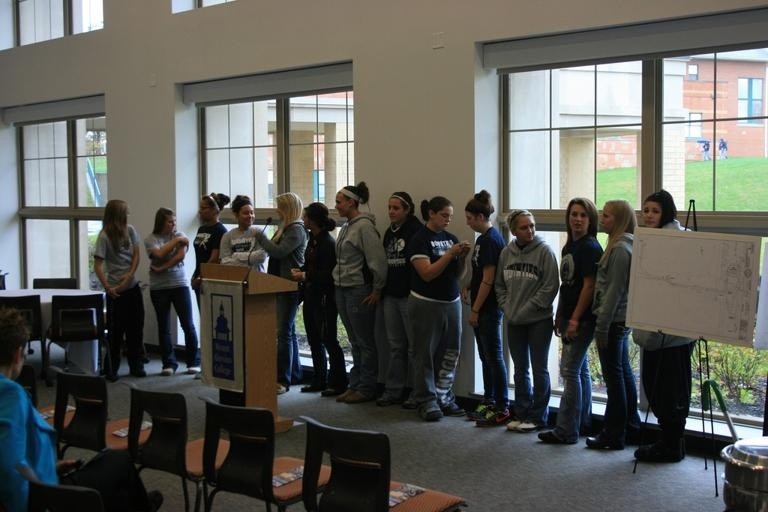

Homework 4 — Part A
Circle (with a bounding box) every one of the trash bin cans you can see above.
[720,436,764,511]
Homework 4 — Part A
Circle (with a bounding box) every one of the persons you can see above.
[191,193,230,312]
[219,195,268,272]
[719,138,728,159]
[333,182,388,404]
[144,207,201,376]
[538,198,603,444]
[405,196,470,421]
[95,200,147,382]
[292,202,347,397]
[586,199,641,451]
[703,141,711,160]
[376,192,424,408]
[632,189,697,463]
[461,189,510,427]
[251,193,308,394]
[495,210,559,434]
[0,307,163,511]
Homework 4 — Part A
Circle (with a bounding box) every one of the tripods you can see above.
[632,202,737,498]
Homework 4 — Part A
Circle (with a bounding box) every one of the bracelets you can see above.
[568,320,579,326]
[471,308,480,314]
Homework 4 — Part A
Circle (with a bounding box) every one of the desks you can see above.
[0,288,106,372]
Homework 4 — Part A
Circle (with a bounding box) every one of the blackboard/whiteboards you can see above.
[624,227,761,349]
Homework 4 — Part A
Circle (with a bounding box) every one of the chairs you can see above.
[33,278,79,290]
[123,381,230,512]
[46,294,117,385]
[0,294,46,379]
[16,365,76,428]
[197,394,331,512]
[55,369,152,460]
[300,415,471,512]
[30,482,105,512]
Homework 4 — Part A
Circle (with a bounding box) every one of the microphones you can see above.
[246,216,272,264]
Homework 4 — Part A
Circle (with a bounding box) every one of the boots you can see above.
[639,441,686,460]
[634,434,682,464]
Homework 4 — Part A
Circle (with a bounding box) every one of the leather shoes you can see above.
[624,439,640,446]
[586,436,605,450]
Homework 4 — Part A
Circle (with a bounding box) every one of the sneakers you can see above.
[105,373,118,379]
[344,393,369,404]
[148,490,163,512]
[321,389,337,397]
[134,370,146,378]
[336,389,355,402]
[476,409,510,427]
[426,410,443,421]
[467,405,488,421]
[276,383,286,394]
[160,368,174,376]
[188,367,201,374]
[507,420,521,431]
[301,384,322,392]
[195,372,201,379]
[449,408,466,417]
[401,401,417,409]
[376,398,394,407]
[538,431,561,443]
[517,421,538,433]
